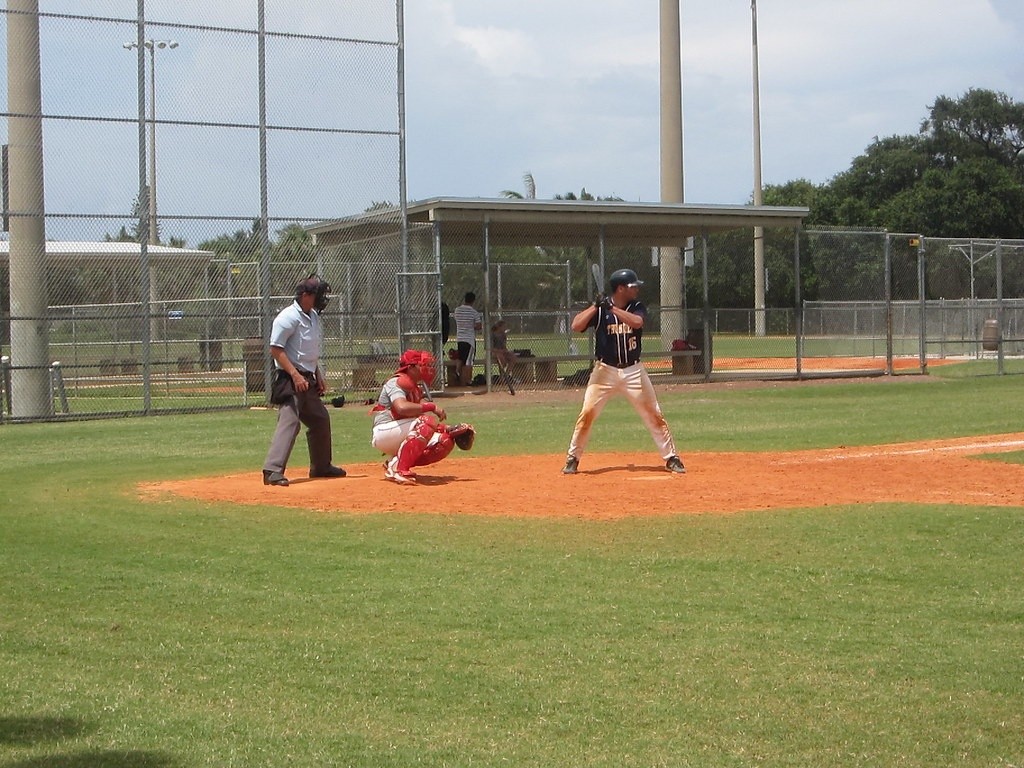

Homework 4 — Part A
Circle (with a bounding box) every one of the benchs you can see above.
[340,349,702,389]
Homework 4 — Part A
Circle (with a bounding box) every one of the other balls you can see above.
[993,320,998,324]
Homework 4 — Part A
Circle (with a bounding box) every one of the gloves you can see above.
[595,292,614,310]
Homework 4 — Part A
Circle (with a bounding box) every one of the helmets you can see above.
[610,269,644,288]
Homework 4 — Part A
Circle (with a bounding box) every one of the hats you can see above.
[296,279,319,294]
[395,350,422,374]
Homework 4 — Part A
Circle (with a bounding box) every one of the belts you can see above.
[597,358,640,368]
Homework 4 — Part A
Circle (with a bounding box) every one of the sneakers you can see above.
[666,456,685,473]
[564,455,578,473]
[382,460,392,468]
[385,468,417,485]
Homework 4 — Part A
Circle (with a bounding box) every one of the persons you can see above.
[490,320,517,386]
[262,278,346,486]
[562,269,686,475]
[373,349,454,486]
[454,292,482,388]
[431,294,450,390]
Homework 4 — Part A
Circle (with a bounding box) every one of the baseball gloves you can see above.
[450,423,476,450]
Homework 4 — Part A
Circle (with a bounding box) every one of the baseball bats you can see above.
[422,379,433,402]
[591,263,605,307]
[493,348,516,395]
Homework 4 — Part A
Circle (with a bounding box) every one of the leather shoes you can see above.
[310,464,346,477]
[263,475,289,486]
